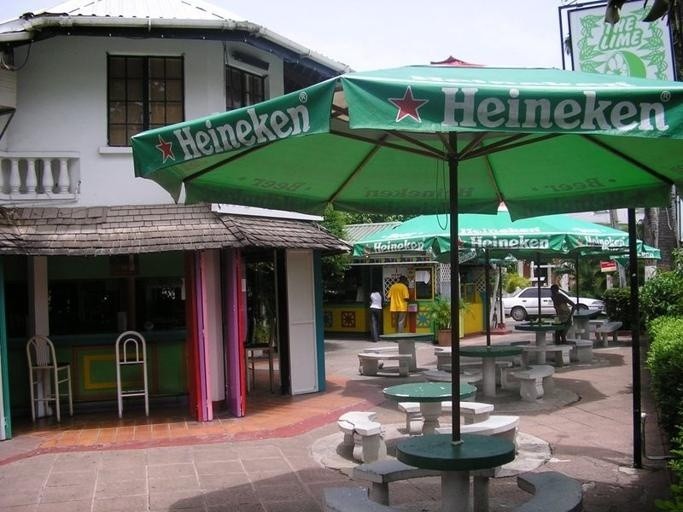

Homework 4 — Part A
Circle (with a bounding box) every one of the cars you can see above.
[496,285,608,325]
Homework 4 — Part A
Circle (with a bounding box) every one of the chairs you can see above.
[244,316,278,393]
[244,316,257,388]
[115,330,150,419]
[26,335,74,423]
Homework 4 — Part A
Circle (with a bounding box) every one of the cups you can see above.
[553,316,559,323]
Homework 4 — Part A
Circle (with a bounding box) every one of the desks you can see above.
[396,433,517,510]
[382,382,477,432]
[459,345,523,397]
[379,332,435,370]
[572,309,602,337]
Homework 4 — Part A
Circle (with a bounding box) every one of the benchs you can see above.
[440,361,513,386]
[337,409,385,463]
[434,414,520,452]
[363,346,417,365]
[397,400,495,435]
[426,369,483,384]
[434,319,624,371]
[357,352,412,377]
[353,459,502,509]
[330,470,583,511]
[511,364,555,400]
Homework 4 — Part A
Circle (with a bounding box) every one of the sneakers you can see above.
[372,337,381,342]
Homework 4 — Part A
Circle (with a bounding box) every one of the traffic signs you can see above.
[600,259,617,272]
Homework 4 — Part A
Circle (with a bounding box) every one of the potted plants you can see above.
[429,293,470,347]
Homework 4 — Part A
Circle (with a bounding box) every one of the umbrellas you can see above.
[130,55,683,446]
